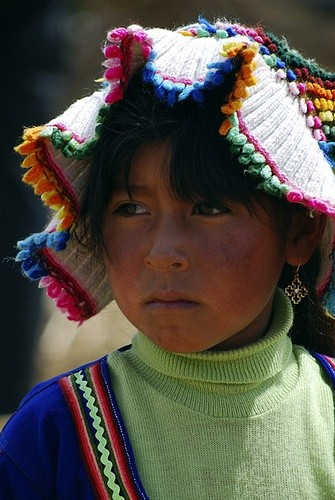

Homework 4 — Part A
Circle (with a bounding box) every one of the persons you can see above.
[0,23,335,499]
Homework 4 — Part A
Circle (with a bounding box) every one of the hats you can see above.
[15,16,335,327]
